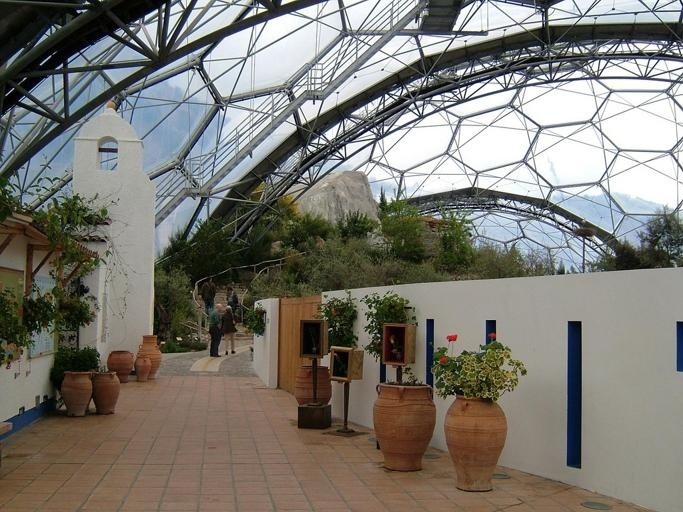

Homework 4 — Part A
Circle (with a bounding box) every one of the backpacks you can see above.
[200,282,213,300]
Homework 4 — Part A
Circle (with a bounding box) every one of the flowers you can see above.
[428,333,527,402]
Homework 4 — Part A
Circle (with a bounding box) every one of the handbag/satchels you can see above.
[227,297,233,306]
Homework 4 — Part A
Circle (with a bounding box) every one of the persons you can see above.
[208,304,224,357]
[197,293,205,310]
[226,287,239,324]
[200,276,216,318]
[221,306,238,355]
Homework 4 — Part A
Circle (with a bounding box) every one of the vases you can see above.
[444,395,507,491]
[61,371,120,417]
[107,335,163,383]
[295,366,332,405]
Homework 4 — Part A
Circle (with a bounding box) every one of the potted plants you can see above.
[373,367,436,472]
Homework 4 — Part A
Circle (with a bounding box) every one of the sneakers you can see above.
[209,350,235,357]
